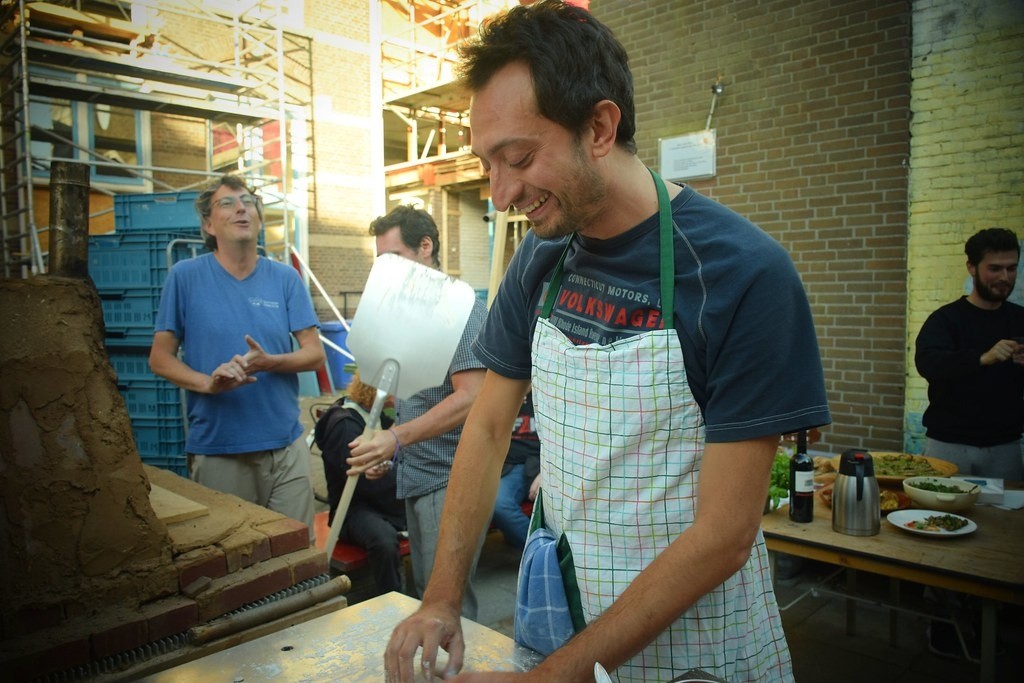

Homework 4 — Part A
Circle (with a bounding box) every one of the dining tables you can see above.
[758,446,1024,683]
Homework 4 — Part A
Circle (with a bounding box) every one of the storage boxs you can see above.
[105,342,185,382]
[142,458,192,480]
[130,418,190,458]
[119,381,186,422]
[96,289,166,341]
[85,234,265,290]
[114,190,265,236]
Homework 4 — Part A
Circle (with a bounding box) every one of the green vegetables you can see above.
[767,444,799,511]
[908,479,969,493]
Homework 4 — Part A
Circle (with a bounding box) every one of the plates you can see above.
[819,485,911,515]
[887,510,977,537]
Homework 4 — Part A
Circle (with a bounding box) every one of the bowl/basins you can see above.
[831,451,959,487]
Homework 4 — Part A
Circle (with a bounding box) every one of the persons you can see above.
[314,367,410,595]
[914,228,1024,664]
[148,170,324,546]
[383,2,832,683]
[491,385,542,551]
[345,203,490,620]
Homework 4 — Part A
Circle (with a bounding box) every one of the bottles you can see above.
[790,429,815,523]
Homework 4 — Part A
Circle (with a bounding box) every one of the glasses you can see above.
[207,195,259,208]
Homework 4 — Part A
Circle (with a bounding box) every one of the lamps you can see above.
[712,84,722,95]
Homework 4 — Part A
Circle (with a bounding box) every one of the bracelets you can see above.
[389,427,402,470]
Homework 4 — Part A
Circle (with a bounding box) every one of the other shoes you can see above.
[971,617,1006,656]
[926,621,963,659]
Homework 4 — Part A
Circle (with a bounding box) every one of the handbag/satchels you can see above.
[305,429,329,502]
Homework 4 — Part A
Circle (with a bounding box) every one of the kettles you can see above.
[833,449,881,536]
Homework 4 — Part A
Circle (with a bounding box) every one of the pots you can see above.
[903,477,981,514]
